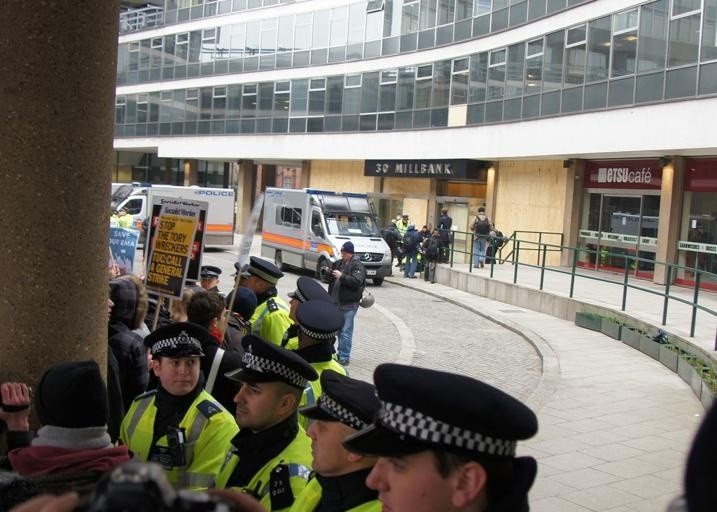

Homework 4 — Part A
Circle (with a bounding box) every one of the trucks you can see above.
[608,212,716,264]
[261,185,393,285]
[111,181,234,253]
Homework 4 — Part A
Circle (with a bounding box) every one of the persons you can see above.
[138,216,150,280]
[318,241,367,365]
[383,213,440,284]
[436,207,452,264]
[484,222,503,264]
[0,255,538,512]
[471,207,493,269]
[109,206,133,230]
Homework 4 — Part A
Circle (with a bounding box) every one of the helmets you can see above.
[357,293,376,309]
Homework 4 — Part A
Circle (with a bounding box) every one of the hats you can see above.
[288,276,329,303]
[295,299,345,341]
[200,265,222,279]
[230,256,284,285]
[223,334,319,390]
[298,369,380,431]
[341,242,354,254]
[143,322,209,358]
[340,363,538,463]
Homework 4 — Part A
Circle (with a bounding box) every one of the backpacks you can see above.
[476,215,489,237]
[492,230,503,247]
[403,235,415,252]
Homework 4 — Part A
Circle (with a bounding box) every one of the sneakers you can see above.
[332,353,350,366]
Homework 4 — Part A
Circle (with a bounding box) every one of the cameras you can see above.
[321,266,332,276]
